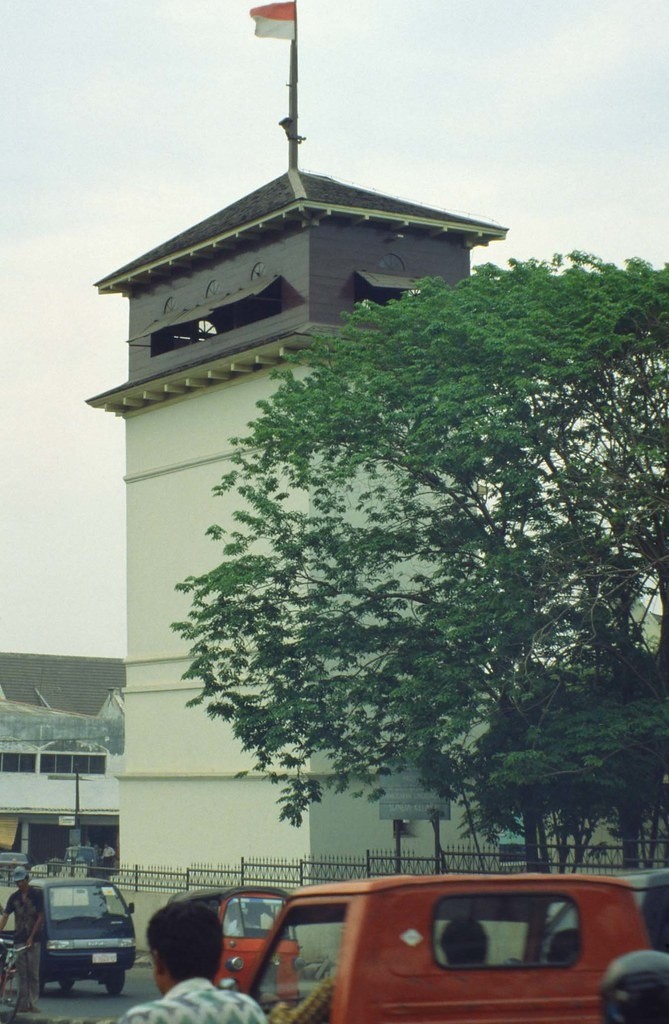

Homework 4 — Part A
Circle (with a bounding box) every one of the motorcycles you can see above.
[540,871,669,966]
[0,876,138,996]
[155,885,300,1013]
[244,874,657,1024]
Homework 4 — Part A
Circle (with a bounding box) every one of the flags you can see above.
[249,0,297,40]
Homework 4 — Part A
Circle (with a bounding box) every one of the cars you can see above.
[63,847,98,876]
[0,852,37,884]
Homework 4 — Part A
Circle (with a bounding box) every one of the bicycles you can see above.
[0,940,37,1024]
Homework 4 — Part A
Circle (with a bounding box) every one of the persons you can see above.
[440,917,490,964]
[86,840,116,867]
[119,900,269,1024]
[0,867,46,1012]
[260,971,334,1024]
[548,926,580,963]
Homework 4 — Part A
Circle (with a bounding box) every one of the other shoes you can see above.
[17,1006,29,1012]
[30,1007,40,1012]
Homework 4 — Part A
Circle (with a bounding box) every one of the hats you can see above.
[13,866,26,881]
